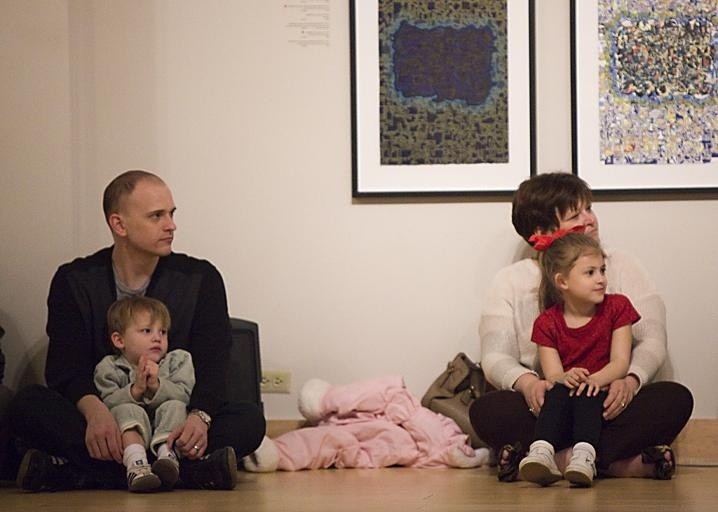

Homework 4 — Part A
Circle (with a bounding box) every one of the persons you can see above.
[519,235,640,485]
[94,295,195,489]
[470,173,694,482]
[10,170,266,493]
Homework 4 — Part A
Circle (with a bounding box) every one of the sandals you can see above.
[497,445,527,482]
[642,445,676,480]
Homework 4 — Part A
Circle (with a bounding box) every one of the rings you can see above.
[194,445,199,450]
[529,408,534,413]
[621,402,625,409]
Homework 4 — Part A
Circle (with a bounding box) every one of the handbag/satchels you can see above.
[422,352,497,449]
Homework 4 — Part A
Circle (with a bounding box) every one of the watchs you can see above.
[186,409,211,428]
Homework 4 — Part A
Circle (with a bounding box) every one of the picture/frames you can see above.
[568,0,718,196]
[347,0,538,198]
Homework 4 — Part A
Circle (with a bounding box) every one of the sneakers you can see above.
[185,448,237,491]
[518,452,563,487]
[17,447,83,493]
[152,454,180,492]
[564,450,597,487]
[127,463,161,494]
